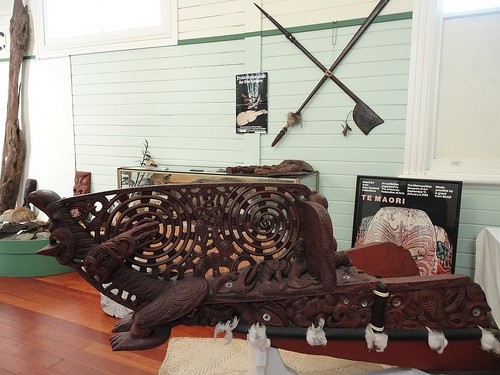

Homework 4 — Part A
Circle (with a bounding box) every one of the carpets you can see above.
[156,335,433,375]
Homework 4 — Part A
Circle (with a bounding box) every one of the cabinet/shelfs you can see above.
[118,168,300,236]
